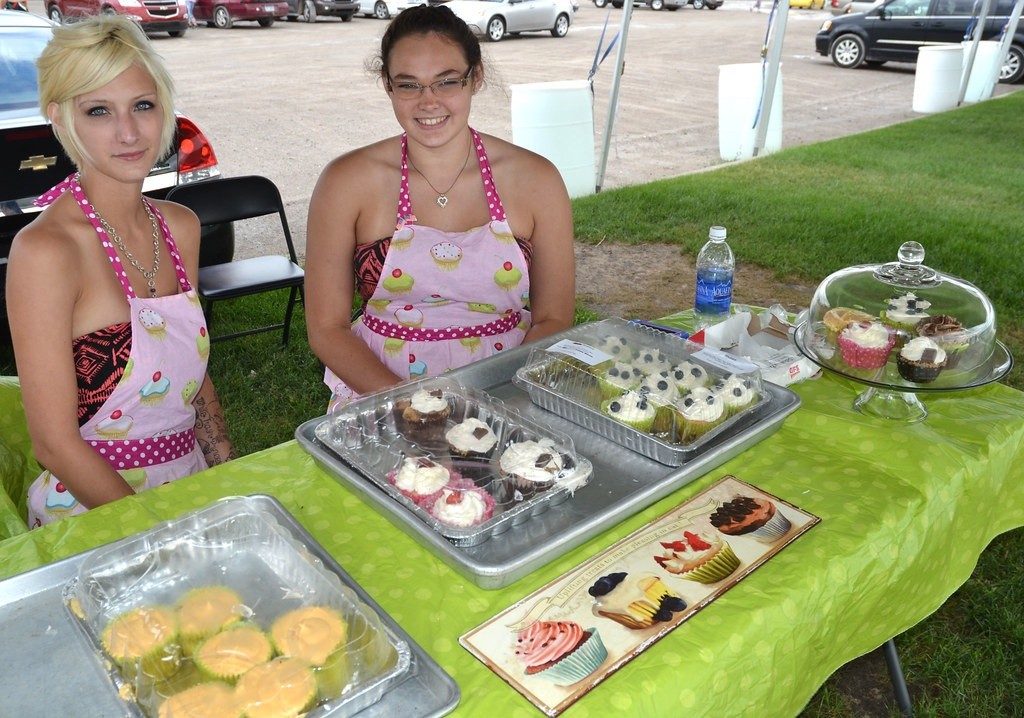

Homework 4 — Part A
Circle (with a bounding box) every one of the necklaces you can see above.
[90,197,161,299]
[407,136,471,210]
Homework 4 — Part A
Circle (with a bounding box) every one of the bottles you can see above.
[692,225,736,333]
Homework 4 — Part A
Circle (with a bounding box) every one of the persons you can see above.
[5,13,238,532]
[302,4,577,417]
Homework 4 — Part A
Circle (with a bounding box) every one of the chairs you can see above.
[165,176,307,346]
[0,376,42,541]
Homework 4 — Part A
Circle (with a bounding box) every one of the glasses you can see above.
[384,66,474,100]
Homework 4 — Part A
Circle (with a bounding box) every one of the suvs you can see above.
[813,0,1024,84]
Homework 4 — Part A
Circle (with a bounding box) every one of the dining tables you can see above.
[0,302,1024,718]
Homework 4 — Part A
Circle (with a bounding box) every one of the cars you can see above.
[1,9,235,322]
[438,0,574,42]
[789,0,886,17]
[43,0,430,38]
[593,0,725,12]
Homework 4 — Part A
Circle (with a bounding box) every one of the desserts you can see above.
[588,572,687,629]
[515,610,608,686]
[101,584,348,718]
[653,530,740,585]
[585,335,760,445]
[710,497,791,544]
[388,388,568,527]
[823,290,970,383]
